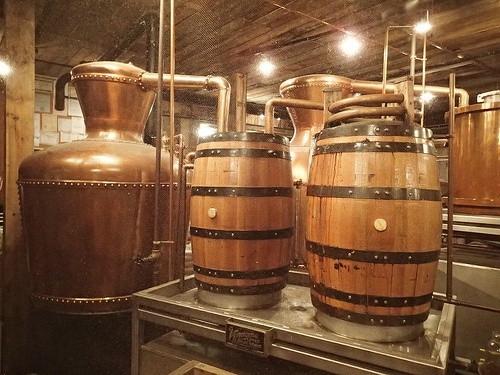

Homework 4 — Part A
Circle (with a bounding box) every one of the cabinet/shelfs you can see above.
[131,289,456,375]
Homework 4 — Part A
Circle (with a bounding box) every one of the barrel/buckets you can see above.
[305,120,443,343]
[190,132,293,309]
